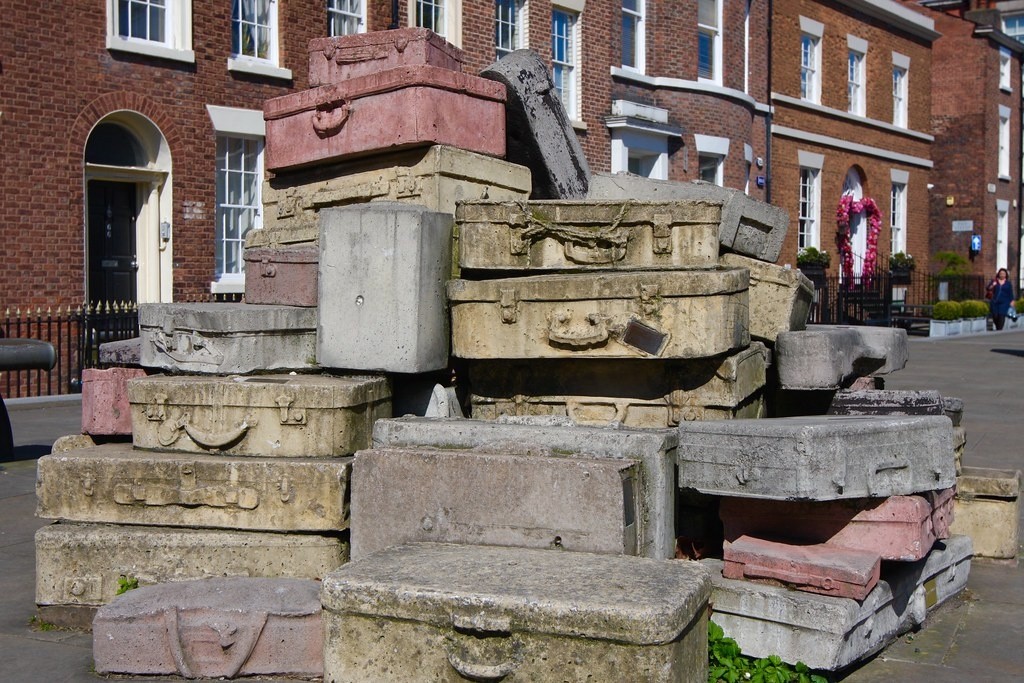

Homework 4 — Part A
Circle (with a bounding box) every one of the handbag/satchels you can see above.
[985,279,998,299]
[1006,306,1021,323]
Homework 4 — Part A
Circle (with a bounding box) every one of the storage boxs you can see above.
[33,25,976,683]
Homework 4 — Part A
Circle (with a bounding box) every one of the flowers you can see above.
[836,194,882,291]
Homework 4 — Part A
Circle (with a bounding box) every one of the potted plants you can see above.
[796,246,831,289]
[889,251,916,285]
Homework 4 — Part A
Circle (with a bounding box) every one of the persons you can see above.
[986,268,1016,331]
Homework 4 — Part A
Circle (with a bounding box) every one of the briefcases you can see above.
[21,26,974,682]
